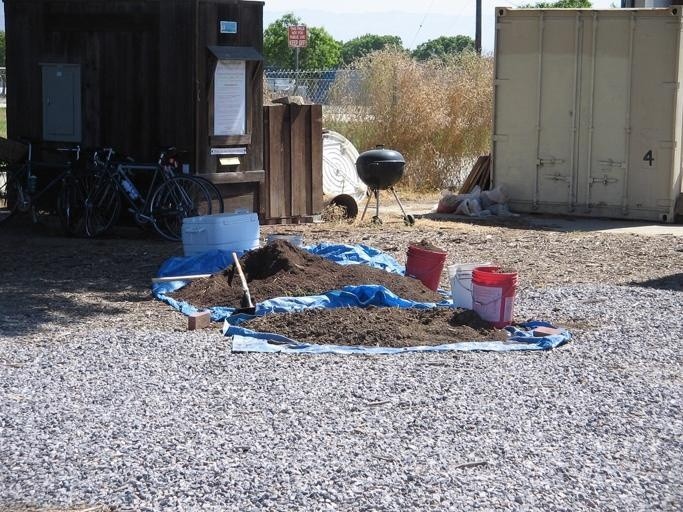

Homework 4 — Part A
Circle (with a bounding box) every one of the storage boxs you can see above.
[181,208,260,258]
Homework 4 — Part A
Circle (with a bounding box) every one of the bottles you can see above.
[29,174,37,192]
[119,178,138,200]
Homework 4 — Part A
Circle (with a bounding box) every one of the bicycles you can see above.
[0,137,222,243]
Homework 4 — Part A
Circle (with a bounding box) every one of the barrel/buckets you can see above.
[471,266,517,330]
[403,242,448,293]
[447,263,490,309]
